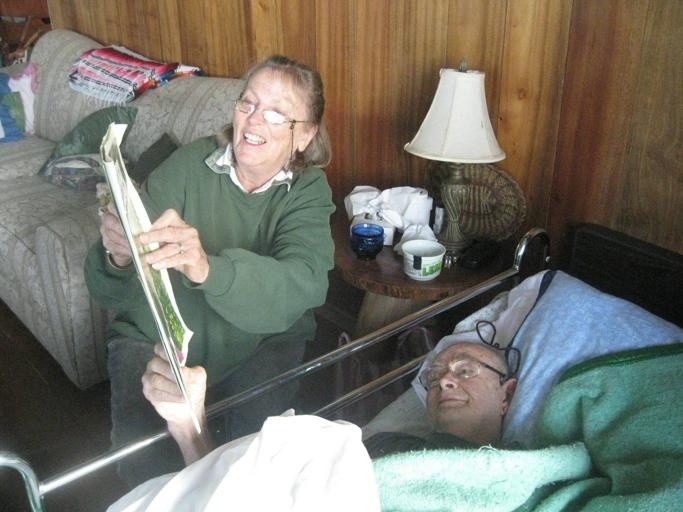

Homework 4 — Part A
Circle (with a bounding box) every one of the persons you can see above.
[81,51,338,486]
[140,336,518,512]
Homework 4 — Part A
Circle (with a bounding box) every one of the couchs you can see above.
[0,27,272,399]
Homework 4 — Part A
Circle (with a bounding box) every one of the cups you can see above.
[401,239,447,282]
[350,223,384,261]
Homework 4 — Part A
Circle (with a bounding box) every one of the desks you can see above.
[322,215,495,361]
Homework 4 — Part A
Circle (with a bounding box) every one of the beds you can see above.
[1,216,681,510]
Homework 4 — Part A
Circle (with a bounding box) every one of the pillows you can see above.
[497,268,682,455]
[42,150,130,190]
[400,269,549,407]
[0,61,37,144]
[34,105,138,181]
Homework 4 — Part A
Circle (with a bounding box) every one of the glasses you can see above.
[229,93,314,128]
[417,348,503,396]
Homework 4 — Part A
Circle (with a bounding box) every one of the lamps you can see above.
[399,57,513,256]
